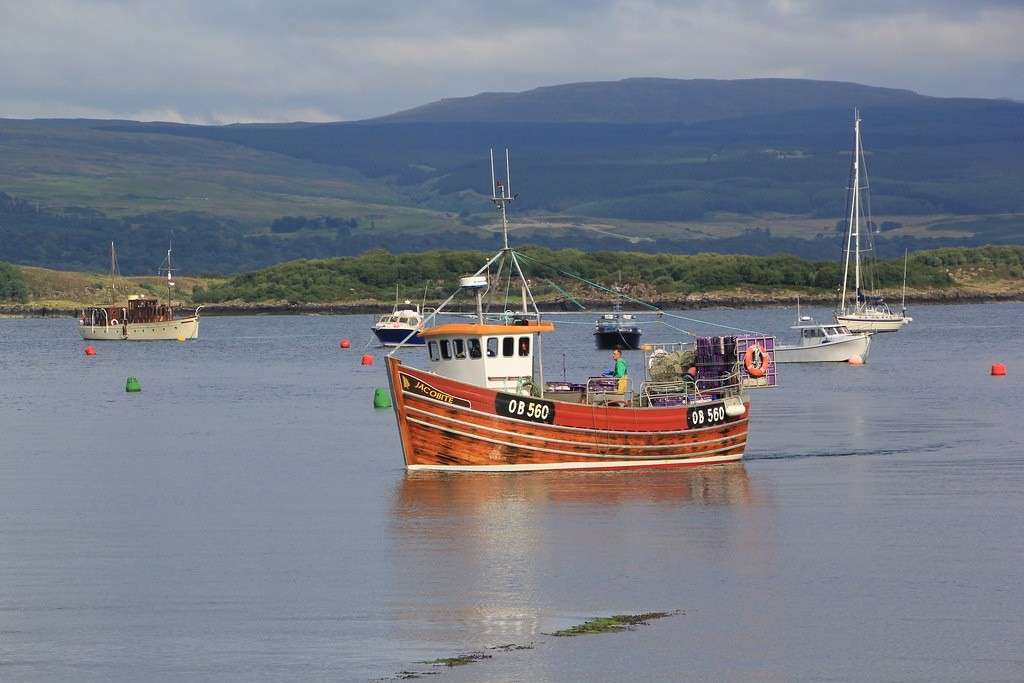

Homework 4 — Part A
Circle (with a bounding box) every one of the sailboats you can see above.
[78,241,203,340]
[371,102,916,471]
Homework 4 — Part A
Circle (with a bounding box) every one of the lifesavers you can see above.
[744,344,769,376]
[111,319,118,326]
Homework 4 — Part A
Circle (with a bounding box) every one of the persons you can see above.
[600,349,628,392]
